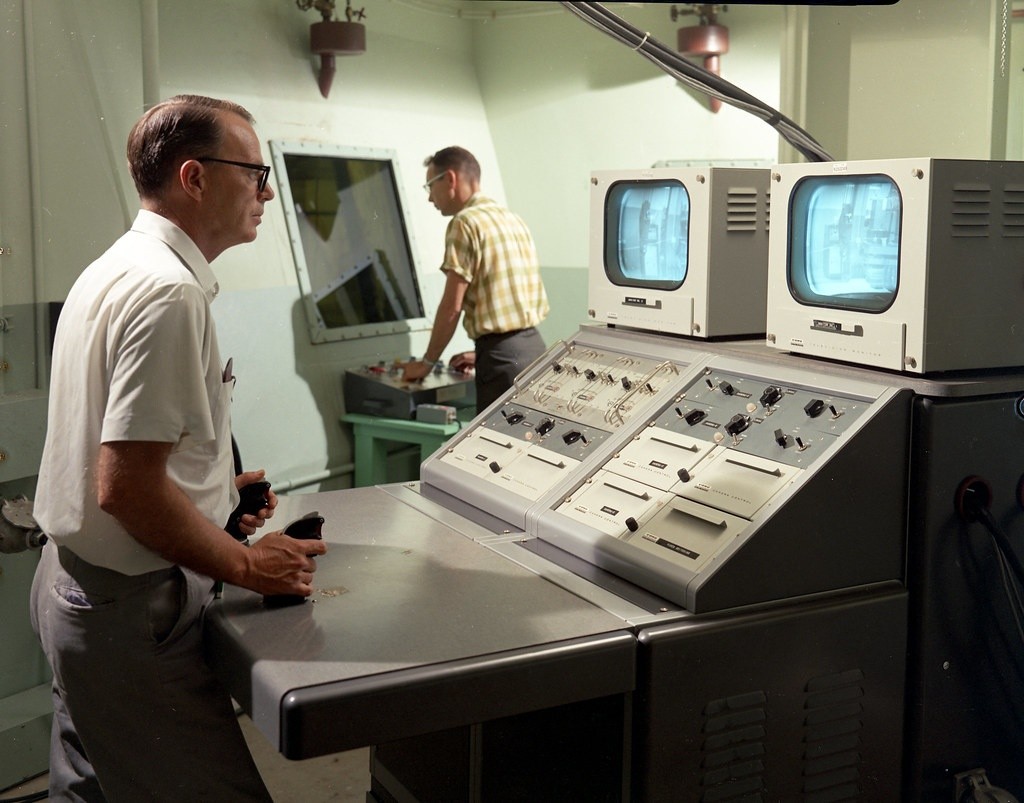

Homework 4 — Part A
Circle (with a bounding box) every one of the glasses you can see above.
[196,156,271,193]
[422,172,447,192]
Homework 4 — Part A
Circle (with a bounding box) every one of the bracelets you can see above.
[423,354,437,367]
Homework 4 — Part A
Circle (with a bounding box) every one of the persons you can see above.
[402,142,553,423]
[23,92,327,803]
